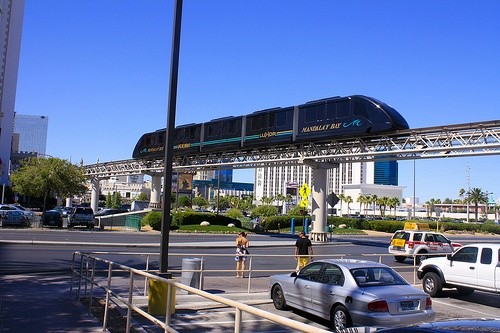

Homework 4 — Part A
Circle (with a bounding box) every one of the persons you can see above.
[235,232,249,278]
[294,231,314,267]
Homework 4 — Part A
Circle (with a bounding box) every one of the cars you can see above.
[268,260,436,333]
[62,207,72,217]
[16,206,26,211]
[39,210,63,227]
[0,205,32,220]
[3,211,29,227]
[372,317,500,333]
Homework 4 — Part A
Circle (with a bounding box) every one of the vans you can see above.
[93,209,118,216]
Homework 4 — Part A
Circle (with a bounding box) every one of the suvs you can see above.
[387,222,462,266]
[67,207,95,229]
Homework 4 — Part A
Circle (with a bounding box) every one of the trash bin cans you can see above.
[182,257,205,296]
[326,226,330,232]
[125,215,142,232]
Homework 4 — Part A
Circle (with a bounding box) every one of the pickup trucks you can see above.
[417,244,500,297]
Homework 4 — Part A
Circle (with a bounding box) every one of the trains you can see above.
[132,93,410,161]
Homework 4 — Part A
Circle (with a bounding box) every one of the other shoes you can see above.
[235,275,240,278]
[242,276,246,278]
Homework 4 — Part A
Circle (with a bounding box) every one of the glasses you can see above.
[239,234,241,235]
